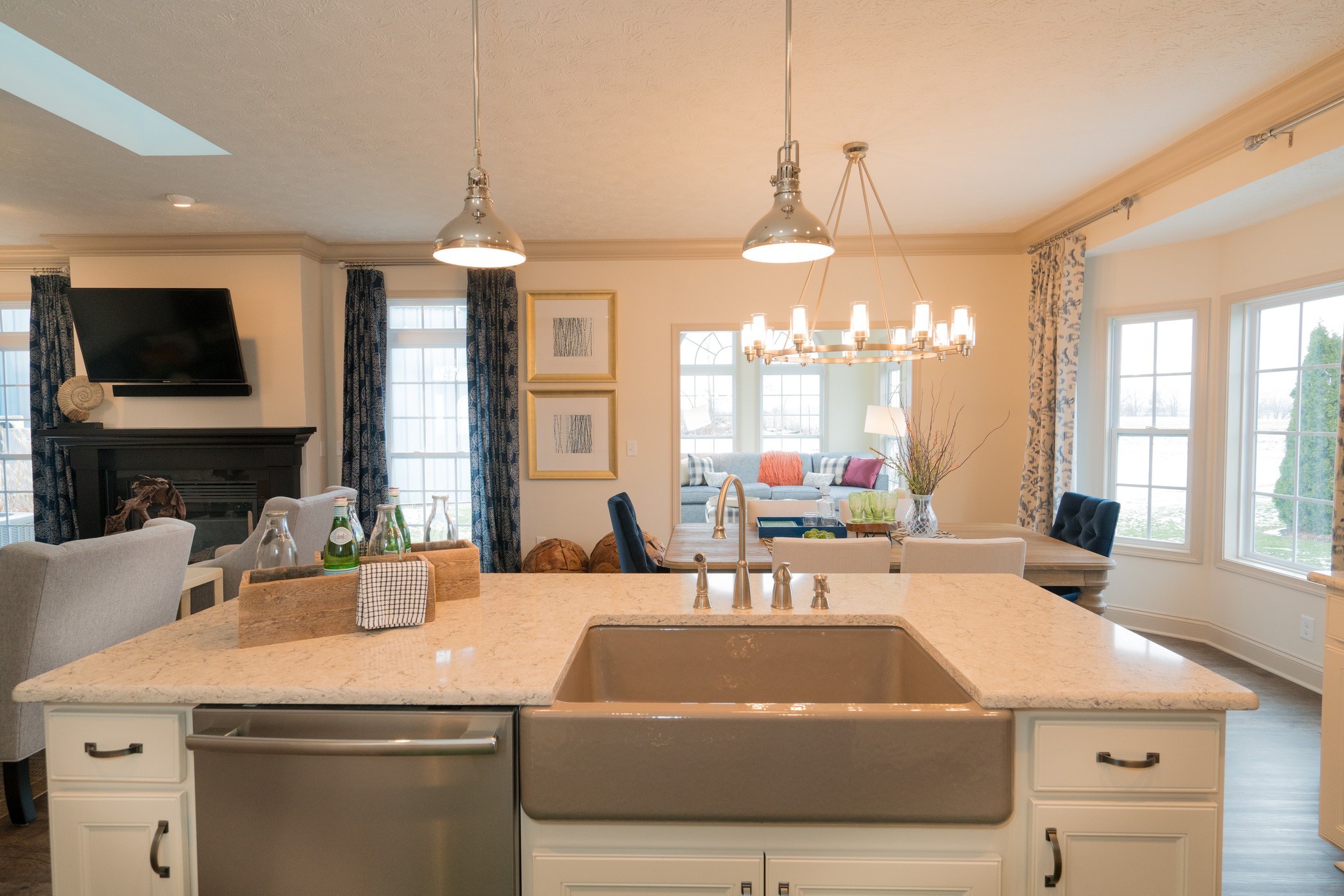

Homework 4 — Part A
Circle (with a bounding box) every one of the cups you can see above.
[861,491,878,520]
[848,492,866,522]
[821,514,835,526]
[802,515,818,526]
[823,516,838,526]
[868,493,887,522]
[803,512,818,515]
[880,491,897,521]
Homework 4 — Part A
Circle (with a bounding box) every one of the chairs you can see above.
[838,498,913,525]
[1040,490,1120,602]
[771,536,891,573]
[746,500,818,524]
[608,491,670,573]
[900,537,1027,578]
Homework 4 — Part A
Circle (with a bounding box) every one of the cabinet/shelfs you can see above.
[42,707,1225,896]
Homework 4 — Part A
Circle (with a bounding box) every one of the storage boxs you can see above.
[238,538,482,649]
[756,517,847,538]
[709,494,760,508]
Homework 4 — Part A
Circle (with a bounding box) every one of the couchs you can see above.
[176,485,359,622]
[0,517,196,826]
[679,452,889,522]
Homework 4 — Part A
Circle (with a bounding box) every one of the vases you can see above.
[903,492,938,539]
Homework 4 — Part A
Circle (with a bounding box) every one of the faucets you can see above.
[710,475,753,610]
[693,552,712,610]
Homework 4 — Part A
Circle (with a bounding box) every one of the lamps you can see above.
[741,140,976,368]
[166,195,195,208]
[432,0,527,269]
[863,377,907,437]
[741,0,836,264]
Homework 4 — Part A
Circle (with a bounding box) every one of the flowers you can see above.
[868,372,1010,534]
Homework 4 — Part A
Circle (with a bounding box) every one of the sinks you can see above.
[518,612,1016,824]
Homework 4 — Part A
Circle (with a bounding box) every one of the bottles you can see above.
[322,497,360,576]
[382,487,412,555]
[255,510,299,582]
[367,504,405,556]
[424,495,458,551]
[347,499,366,557]
[816,485,835,526]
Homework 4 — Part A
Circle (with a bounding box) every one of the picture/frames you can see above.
[526,290,617,482]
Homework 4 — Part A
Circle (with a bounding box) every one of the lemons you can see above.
[804,528,836,539]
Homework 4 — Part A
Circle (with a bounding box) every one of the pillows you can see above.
[680,454,888,492]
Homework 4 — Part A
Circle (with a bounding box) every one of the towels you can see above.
[354,559,429,631]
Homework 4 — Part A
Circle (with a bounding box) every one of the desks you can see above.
[662,522,1117,616]
[704,500,740,523]
[179,567,224,617]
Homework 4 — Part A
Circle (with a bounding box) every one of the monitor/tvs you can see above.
[65,287,247,384]
